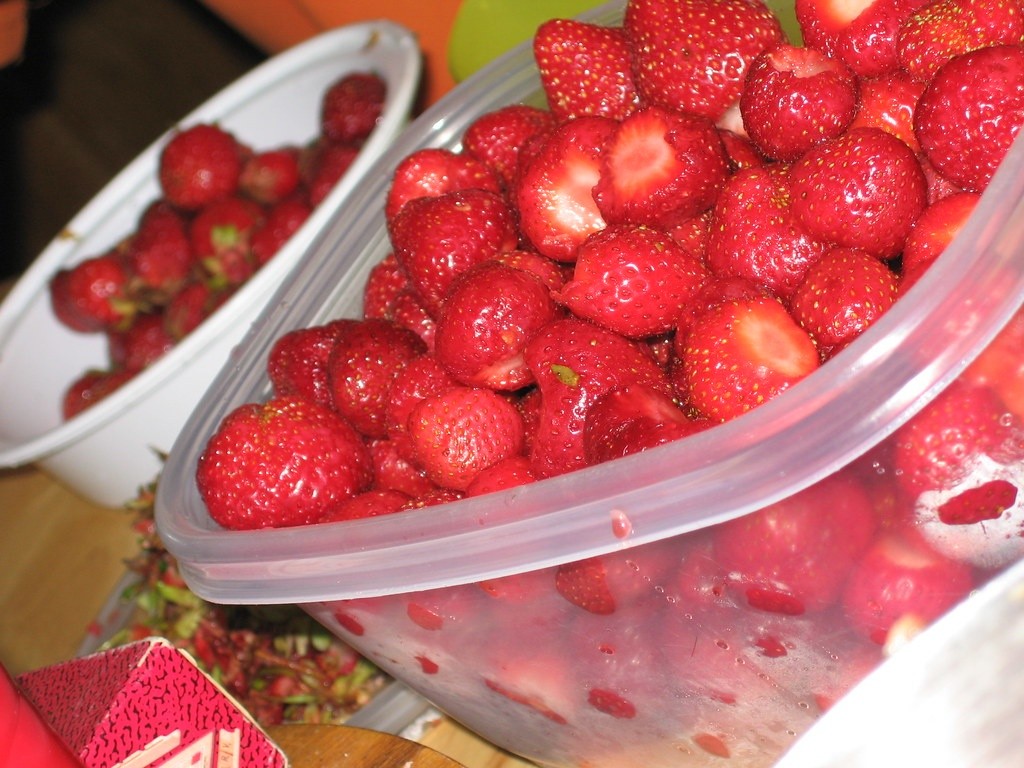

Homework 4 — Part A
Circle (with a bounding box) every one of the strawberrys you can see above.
[48,1,1024,764]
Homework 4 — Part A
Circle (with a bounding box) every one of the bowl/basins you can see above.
[153,2,1024,768]
[1,17,426,512]
